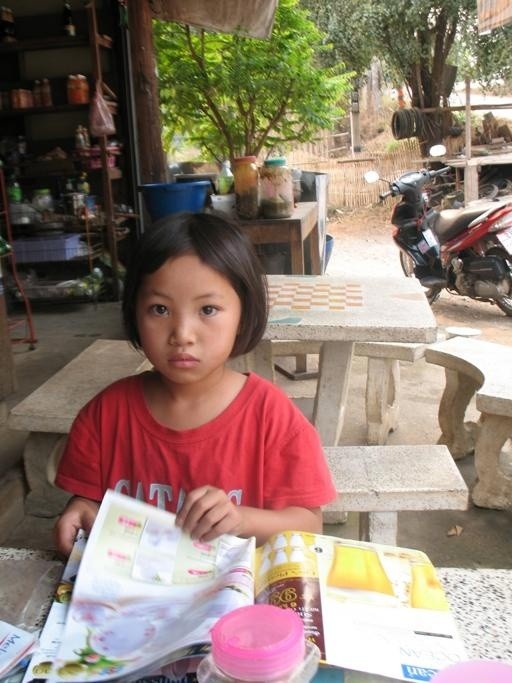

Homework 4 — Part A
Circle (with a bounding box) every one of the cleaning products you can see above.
[215,158,232,193]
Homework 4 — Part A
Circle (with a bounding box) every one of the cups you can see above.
[408,557,449,612]
[326,541,398,596]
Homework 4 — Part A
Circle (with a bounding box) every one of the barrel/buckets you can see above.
[210,194,237,217]
[325,234,335,274]
[137,180,211,224]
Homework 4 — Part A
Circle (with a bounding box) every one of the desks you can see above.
[235,201,320,274]
[0,547,512,683]
[225,274,437,446]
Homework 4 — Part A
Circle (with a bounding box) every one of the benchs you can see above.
[10,337,152,519]
[276,342,434,442]
[332,447,468,550]
[431,336,509,514]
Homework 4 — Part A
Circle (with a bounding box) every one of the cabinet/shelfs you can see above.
[0,0,140,302]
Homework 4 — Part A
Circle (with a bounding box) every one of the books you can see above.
[20,488,469,683]
[0,621,37,681]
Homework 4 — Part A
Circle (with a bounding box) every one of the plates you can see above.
[88,616,159,659]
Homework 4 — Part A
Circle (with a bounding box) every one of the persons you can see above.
[53,212,338,559]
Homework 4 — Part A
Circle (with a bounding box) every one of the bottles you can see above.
[77,171,90,194]
[5,174,23,205]
[65,178,75,194]
[31,188,54,213]
[216,160,235,193]
[33,78,52,106]
[196,603,321,683]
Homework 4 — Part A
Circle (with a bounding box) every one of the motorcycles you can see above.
[363,142,511,318]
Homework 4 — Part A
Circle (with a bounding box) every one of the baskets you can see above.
[70,145,120,169]
[12,280,103,302]
